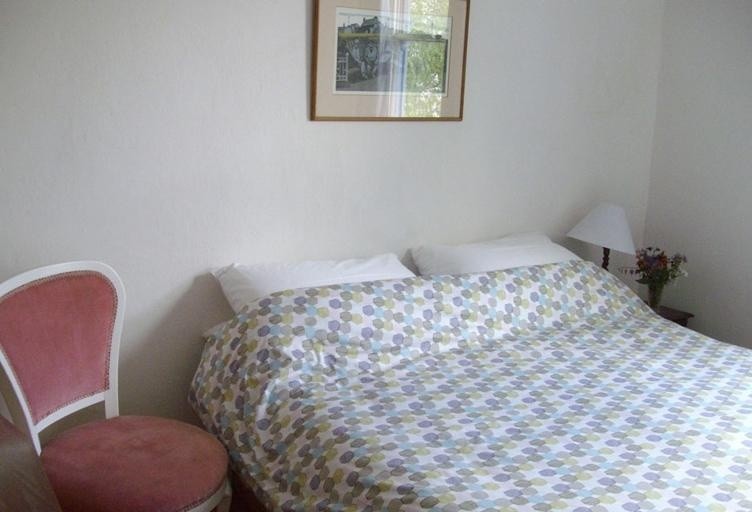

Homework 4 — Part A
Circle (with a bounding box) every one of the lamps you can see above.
[566,202,637,272]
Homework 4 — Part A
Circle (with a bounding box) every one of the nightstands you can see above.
[644,300,695,327]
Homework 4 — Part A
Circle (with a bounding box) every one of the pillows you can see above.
[202,252,419,314]
[410,229,584,277]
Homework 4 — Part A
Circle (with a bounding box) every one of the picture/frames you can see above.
[311,0,470,121]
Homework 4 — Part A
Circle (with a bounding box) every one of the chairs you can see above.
[0,260,234,512]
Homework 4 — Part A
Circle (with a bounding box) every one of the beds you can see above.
[186,260,752,512]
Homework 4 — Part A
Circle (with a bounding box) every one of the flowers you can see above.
[616,246,689,309]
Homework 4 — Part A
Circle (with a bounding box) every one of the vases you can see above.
[647,283,665,314]
